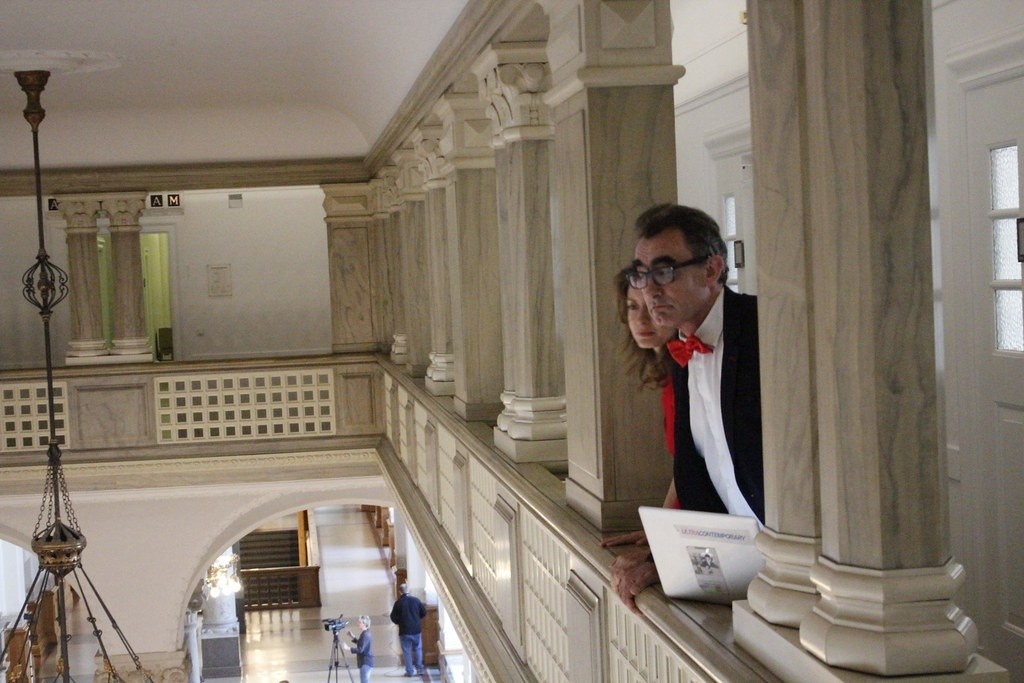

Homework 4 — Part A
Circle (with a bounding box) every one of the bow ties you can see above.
[665,333,714,368]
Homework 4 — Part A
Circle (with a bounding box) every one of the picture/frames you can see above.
[734,240,744,268]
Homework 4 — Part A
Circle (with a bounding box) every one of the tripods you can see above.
[328,629,354,683]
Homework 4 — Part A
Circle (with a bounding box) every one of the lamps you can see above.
[1,70,158,683]
[207,551,242,599]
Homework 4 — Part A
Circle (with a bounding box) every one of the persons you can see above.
[339,615,377,683]
[388,581,430,678]
[600,201,767,616]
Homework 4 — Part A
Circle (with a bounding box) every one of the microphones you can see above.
[322,618,331,622]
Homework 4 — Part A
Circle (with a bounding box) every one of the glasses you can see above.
[623,254,706,290]
